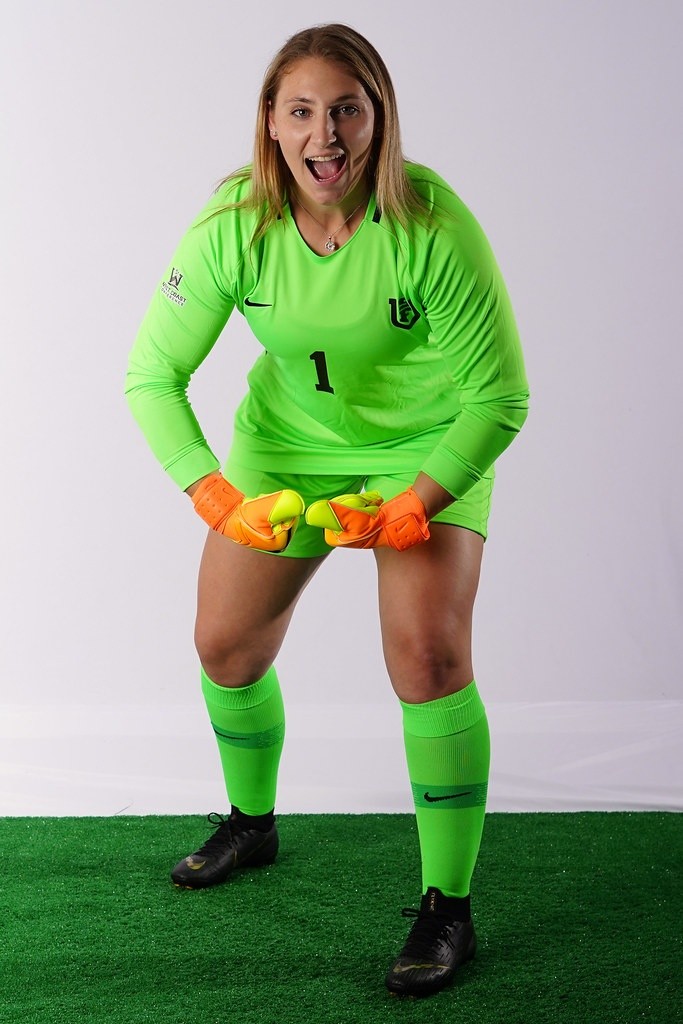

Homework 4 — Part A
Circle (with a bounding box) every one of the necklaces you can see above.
[289,184,372,251]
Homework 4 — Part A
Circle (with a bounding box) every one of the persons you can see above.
[123,23,530,998]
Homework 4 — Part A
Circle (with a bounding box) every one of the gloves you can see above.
[191,473,304,554]
[304,490,430,552]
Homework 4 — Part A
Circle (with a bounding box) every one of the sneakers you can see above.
[170,812,279,889]
[383,886,476,1000]
